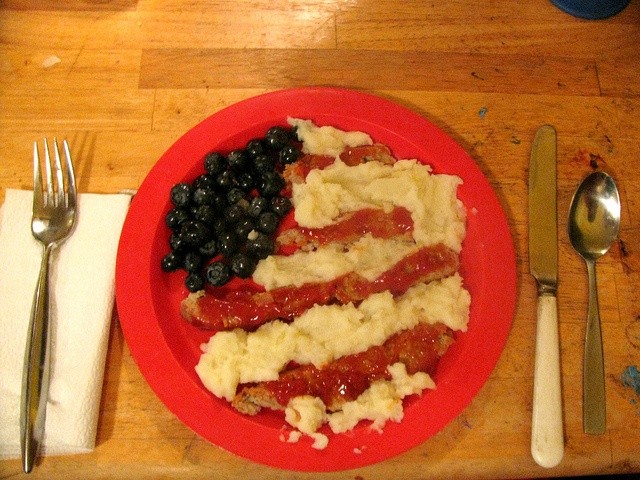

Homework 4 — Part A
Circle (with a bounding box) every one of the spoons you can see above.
[567,169,622,438]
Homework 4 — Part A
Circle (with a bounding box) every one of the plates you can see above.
[114,85,515,472]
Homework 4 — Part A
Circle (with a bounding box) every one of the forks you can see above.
[20,134,79,475]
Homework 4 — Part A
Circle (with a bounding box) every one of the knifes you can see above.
[528,123,566,469]
